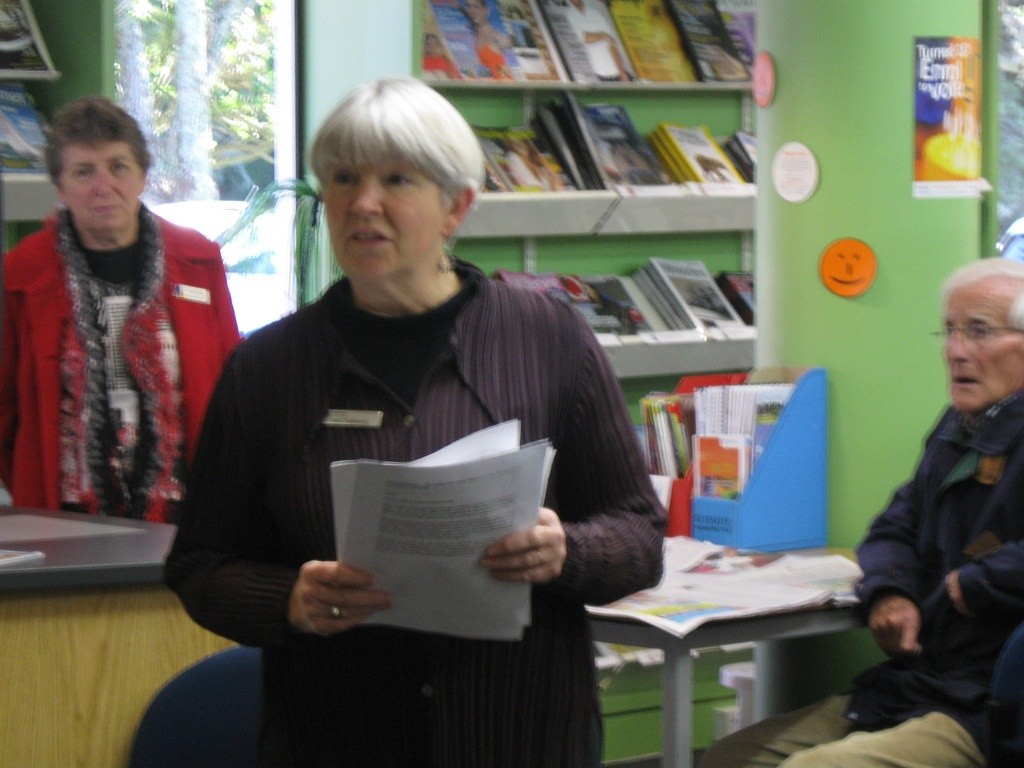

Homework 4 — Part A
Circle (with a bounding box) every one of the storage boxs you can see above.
[655,366,829,553]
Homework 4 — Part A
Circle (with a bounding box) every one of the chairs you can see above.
[127,650,265,768]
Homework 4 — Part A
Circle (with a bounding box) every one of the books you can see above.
[495,258,755,336]
[472,92,756,193]
[422,0,757,83]
[0,0,56,172]
[640,382,797,499]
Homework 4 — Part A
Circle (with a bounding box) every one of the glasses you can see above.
[930,324,1024,344]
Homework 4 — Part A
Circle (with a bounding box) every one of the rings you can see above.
[535,549,543,564]
[330,605,342,617]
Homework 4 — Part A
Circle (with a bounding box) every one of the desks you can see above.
[586,546,869,766]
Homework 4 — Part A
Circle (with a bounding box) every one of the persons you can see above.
[505,134,558,190]
[585,283,635,335]
[161,78,669,767]
[423,34,462,79]
[699,257,1024,768]
[449,0,514,78]
[566,0,632,81]
[0,100,241,526]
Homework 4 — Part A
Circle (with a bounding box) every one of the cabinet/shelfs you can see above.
[411,0,780,380]
